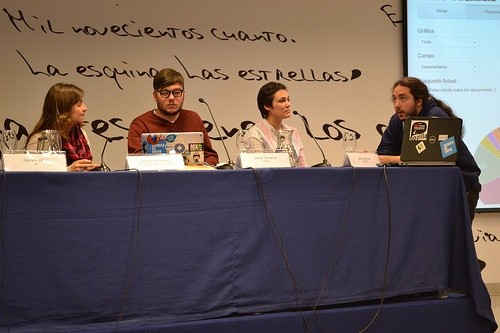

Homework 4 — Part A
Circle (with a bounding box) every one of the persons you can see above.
[127,68,220,167]
[244,82,307,168]
[374,77,482,227]
[24,82,101,173]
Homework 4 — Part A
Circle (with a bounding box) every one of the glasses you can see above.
[156,87,184,98]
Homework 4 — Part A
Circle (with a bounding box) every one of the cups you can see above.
[343,131,357,153]
[236,129,251,153]
[1,129,18,154]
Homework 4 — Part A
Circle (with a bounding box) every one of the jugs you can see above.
[274,129,298,168]
[42,130,63,151]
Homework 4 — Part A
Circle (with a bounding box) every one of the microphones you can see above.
[198,98,237,170]
[292,111,332,167]
[90,130,114,171]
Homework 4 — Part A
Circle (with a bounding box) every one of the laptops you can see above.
[380,115,464,166]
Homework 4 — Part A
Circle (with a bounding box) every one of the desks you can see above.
[0,167,498,333]
